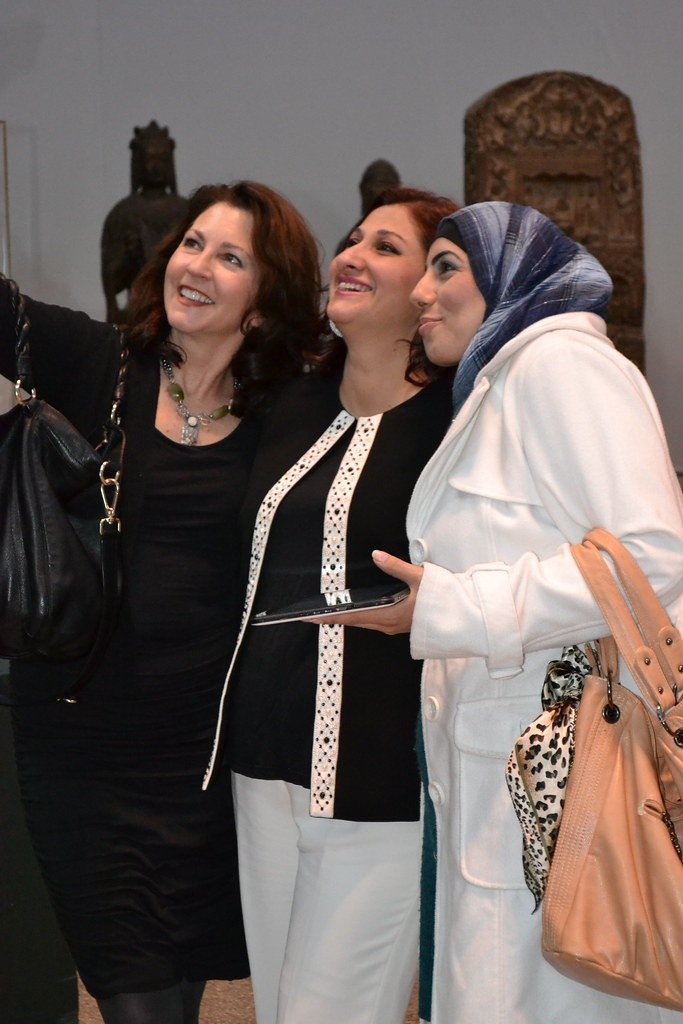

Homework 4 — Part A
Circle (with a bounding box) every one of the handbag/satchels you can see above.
[541,528,683,1012]
[0,280,131,660]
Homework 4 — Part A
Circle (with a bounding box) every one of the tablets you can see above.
[250,581,412,625]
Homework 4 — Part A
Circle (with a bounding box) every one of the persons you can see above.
[0,180,683,1024]
[101,119,188,321]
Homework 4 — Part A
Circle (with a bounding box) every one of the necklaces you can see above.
[161,359,241,446]
